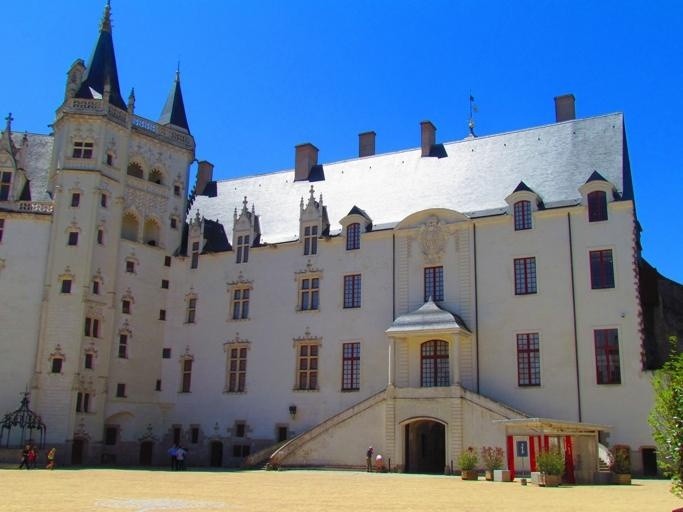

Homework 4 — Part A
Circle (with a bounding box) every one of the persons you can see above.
[167,442,188,470]
[18,443,55,469]
[366,446,373,472]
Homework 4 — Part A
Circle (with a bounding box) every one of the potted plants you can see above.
[479,446,505,480]
[456,450,480,480]
[606,444,632,485]
[536,444,569,487]
